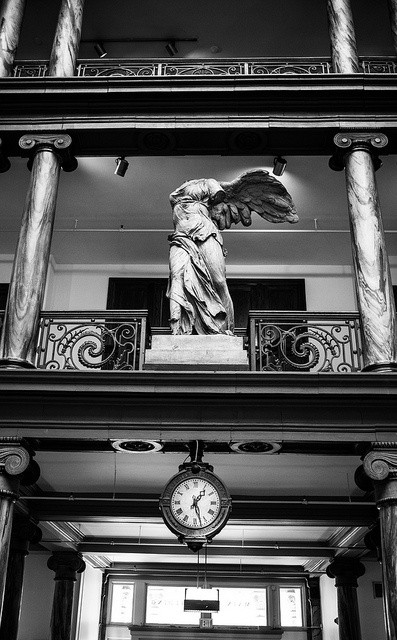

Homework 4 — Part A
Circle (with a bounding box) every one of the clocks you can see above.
[159,465,233,549]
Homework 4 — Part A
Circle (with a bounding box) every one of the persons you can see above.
[166,169,300,335]
